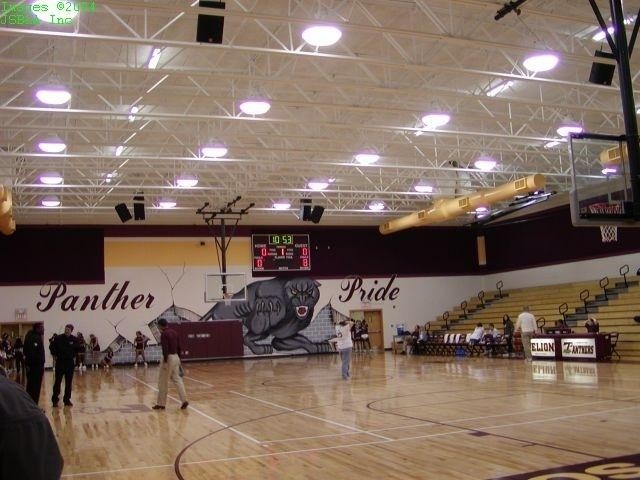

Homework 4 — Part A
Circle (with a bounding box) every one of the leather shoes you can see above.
[181,401,189,409]
[152,405,165,409]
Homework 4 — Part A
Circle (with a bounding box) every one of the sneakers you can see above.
[53,402,59,407]
[64,401,73,406]
[134,363,138,367]
[144,362,148,366]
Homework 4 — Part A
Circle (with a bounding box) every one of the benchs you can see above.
[400,269,637,363]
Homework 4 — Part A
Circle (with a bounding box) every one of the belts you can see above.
[168,352,178,354]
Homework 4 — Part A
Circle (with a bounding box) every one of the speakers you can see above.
[195,0,225,44]
[133,195,145,220]
[114,202,132,224]
[588,49,617,86]
[303,205,311,221]
[311,205,325,224]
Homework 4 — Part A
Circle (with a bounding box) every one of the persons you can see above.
[0,322,114,480]
[400,325,420,355]
[335,320,354,380]
[556,319,569,329]
[415,325,428,355]
[469,314,514,359]
[513,304,539,361]
[585,317,599,333]
[350,318,373,352]
[152,319,189,409]
[133,331,148,366]
